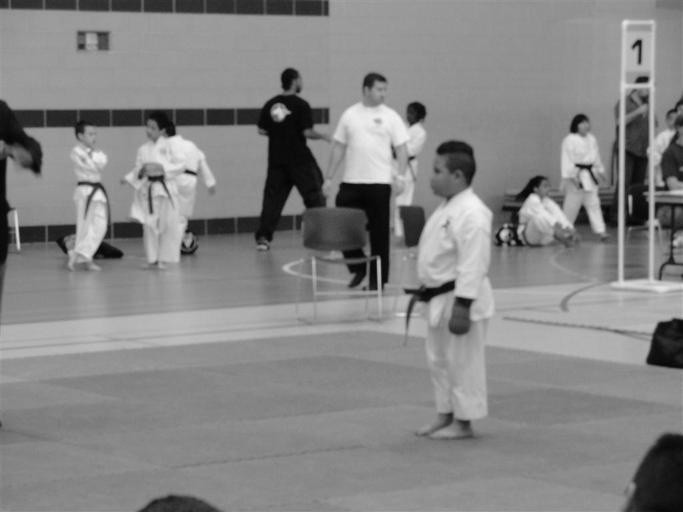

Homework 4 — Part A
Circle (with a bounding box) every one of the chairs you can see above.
[293,206,383,324]
[392,205,427,318]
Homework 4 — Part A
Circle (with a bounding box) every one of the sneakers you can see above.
[257,237,269,250]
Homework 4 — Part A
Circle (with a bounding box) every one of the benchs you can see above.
[503,186,617,230]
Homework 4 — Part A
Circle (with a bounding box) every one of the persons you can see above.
[325,73,408,288]
[391,101,428,248]
[510,172,583,249]
[63,119,108,273]
[253,65,331,251]
[559,114,613,242]
[162,120,219,258]
[612,74,682,253]
[120,109,190,269]
[402,142,498,445]
[0,101,42,291]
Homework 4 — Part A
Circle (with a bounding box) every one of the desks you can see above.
[641,190,682,280]
